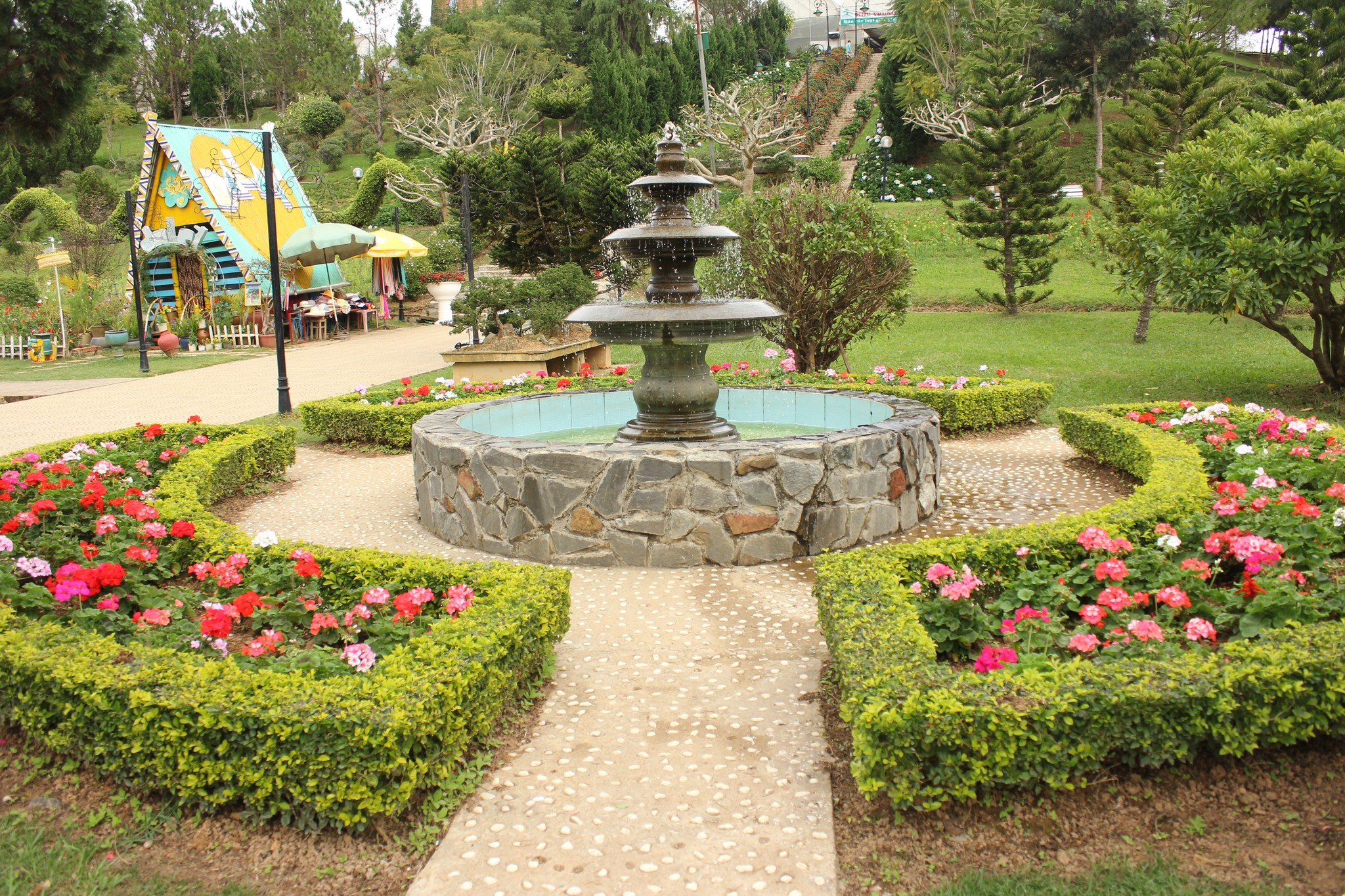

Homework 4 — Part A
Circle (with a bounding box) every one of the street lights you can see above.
[353,167,363,194]
[813,0,833,55]
[855,0,871,56]
[754,48,778,127]
[880,135,892,202]
[805,43,825,124]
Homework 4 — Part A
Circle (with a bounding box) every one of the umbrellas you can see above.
[277,222,376,333]
[349,228,429,331]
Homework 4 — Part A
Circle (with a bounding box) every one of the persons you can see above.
[776,84,788,106]
[825,46,831,56]
[845,40,853,54]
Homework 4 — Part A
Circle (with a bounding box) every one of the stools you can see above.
[311,319,327,342]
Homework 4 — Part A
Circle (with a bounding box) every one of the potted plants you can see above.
[209,255,303,348]
[156,312,168,332]
[439,263,611,385]
[195,313,206,329]
[188,330,199,352]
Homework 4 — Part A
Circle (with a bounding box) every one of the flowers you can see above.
[419,270,466,285]
[198,337,233,347]
[160,304,178,313]
[123,317,145,341]
[168,317,198,337]
[93,299,133,331]
[198,294,225,321]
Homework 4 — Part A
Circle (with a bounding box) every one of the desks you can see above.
[350,309,378,334]
[303,309,359,336]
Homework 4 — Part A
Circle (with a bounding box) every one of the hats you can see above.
[295,288,377,318]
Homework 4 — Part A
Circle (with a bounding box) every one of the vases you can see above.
[105,329,129,360]
[179,337,190,350]
[123,340,140,351]
[165,310,178,324]
[199,343,232,351]
